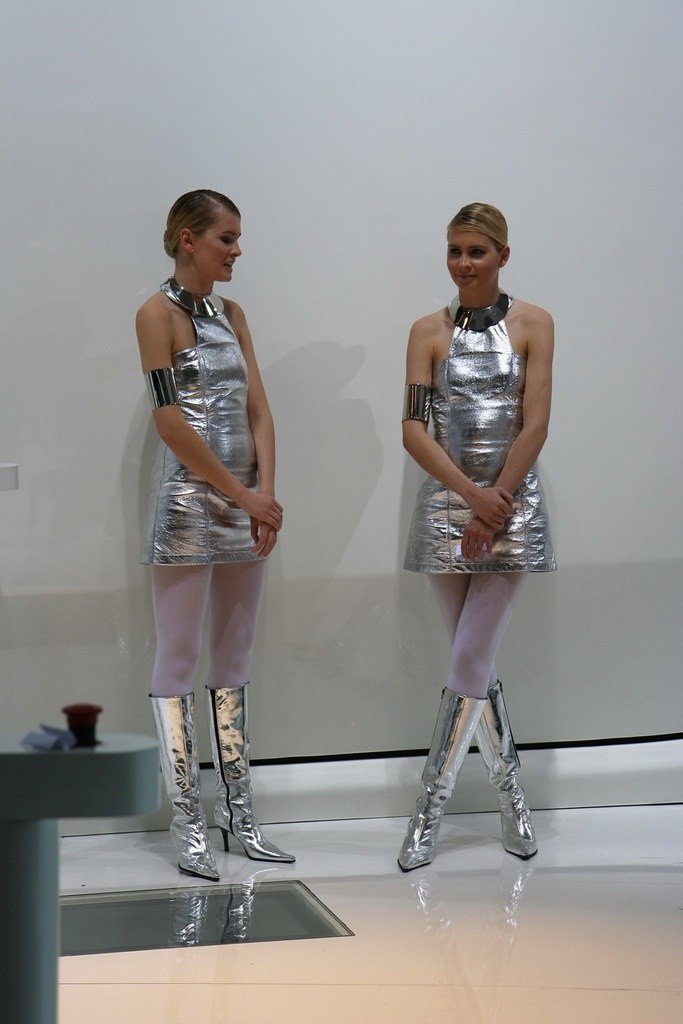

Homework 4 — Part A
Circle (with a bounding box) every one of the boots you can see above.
[475,679,538,860]
[149,691,221,882]
[397,686,488,872]
[205,681,296,863]
